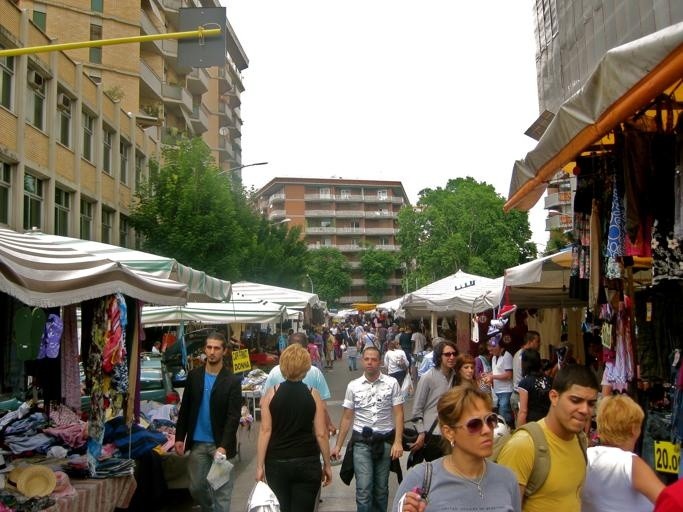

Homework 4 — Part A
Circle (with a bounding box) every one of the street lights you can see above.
[213,162,267,179]
[270,218,292,225]
[305,273,313,294]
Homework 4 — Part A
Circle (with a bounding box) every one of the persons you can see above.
[307,314,428,386]
[330,347,403,512]
[256,333,337,511]
[176,333,241,511]
[388,363,682,512]
[411,331,574,460]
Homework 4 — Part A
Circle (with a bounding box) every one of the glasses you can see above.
[442,352,459,357]
[452,413,498,435]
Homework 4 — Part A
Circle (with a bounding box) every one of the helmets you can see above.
[401,428,419,451]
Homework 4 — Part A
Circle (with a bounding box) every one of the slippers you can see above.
[13,305,63,359]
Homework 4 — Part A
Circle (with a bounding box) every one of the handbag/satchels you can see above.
[399,358,406,369]
[407,443,424,471]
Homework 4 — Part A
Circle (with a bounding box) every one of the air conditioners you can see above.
[56,93,71,112]
[26,70,43,90]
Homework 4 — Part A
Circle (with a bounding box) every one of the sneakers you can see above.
[500,304,517,316]
[353,368,358,371]
[349,366,352,371]
[489,333,502,347]
[324,365,333,368]
[487,318,508,335]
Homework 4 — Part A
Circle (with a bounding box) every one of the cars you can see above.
[0,327,218,415]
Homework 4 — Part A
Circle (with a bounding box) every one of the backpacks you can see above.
[490,421,551,497]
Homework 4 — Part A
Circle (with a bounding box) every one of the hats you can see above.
[17,464,56,497]
[0,453,26,490]
[52,470,75,497]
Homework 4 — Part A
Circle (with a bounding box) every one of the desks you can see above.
[0,352,273,511]
